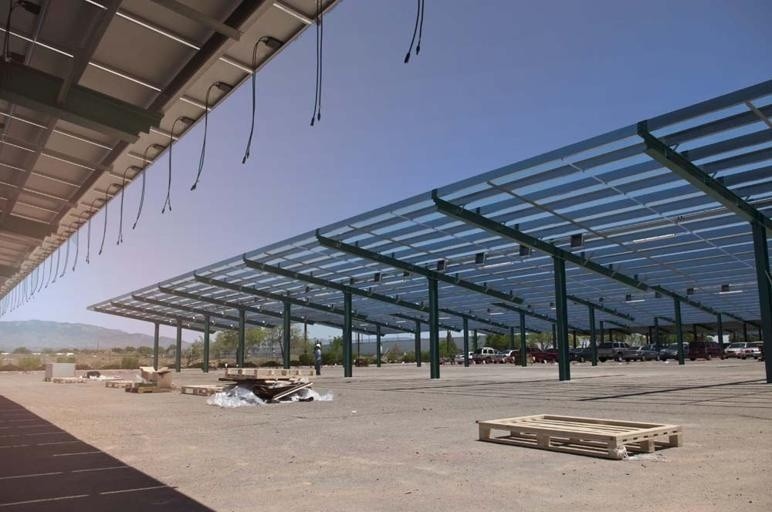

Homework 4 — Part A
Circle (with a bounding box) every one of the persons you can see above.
[312,343,321,374]
